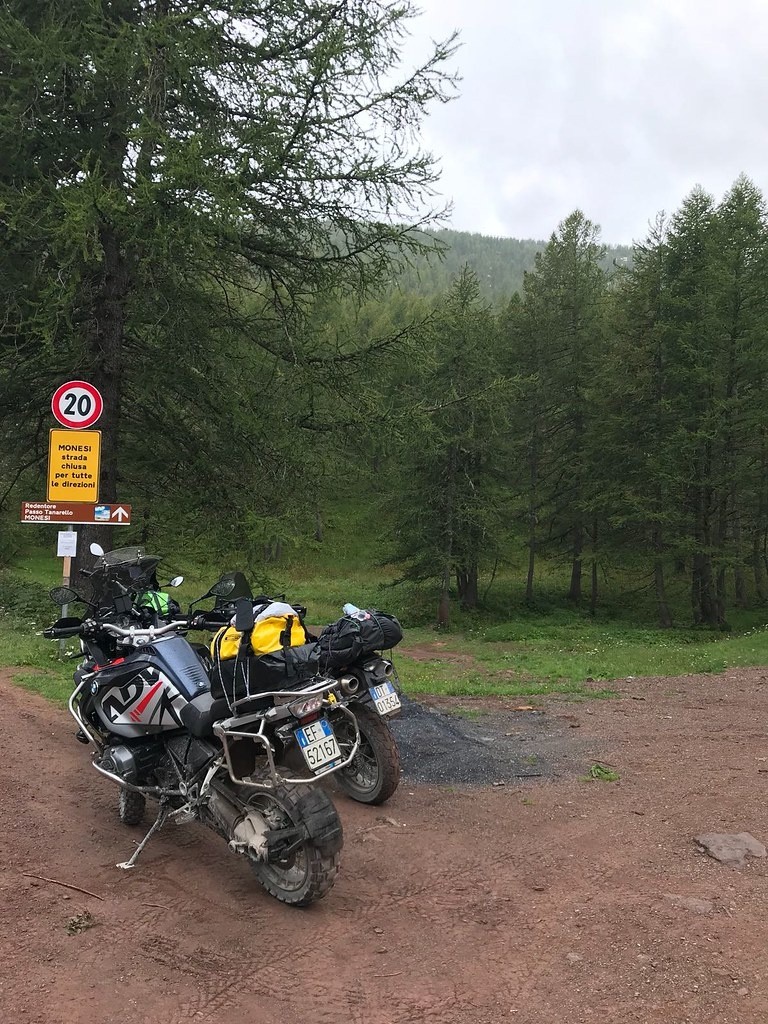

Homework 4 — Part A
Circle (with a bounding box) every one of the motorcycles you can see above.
[40,539,402,909]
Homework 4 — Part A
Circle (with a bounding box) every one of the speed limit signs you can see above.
[51,380,104,430]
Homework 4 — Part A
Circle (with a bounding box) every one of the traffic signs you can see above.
[20,499,132,527]
